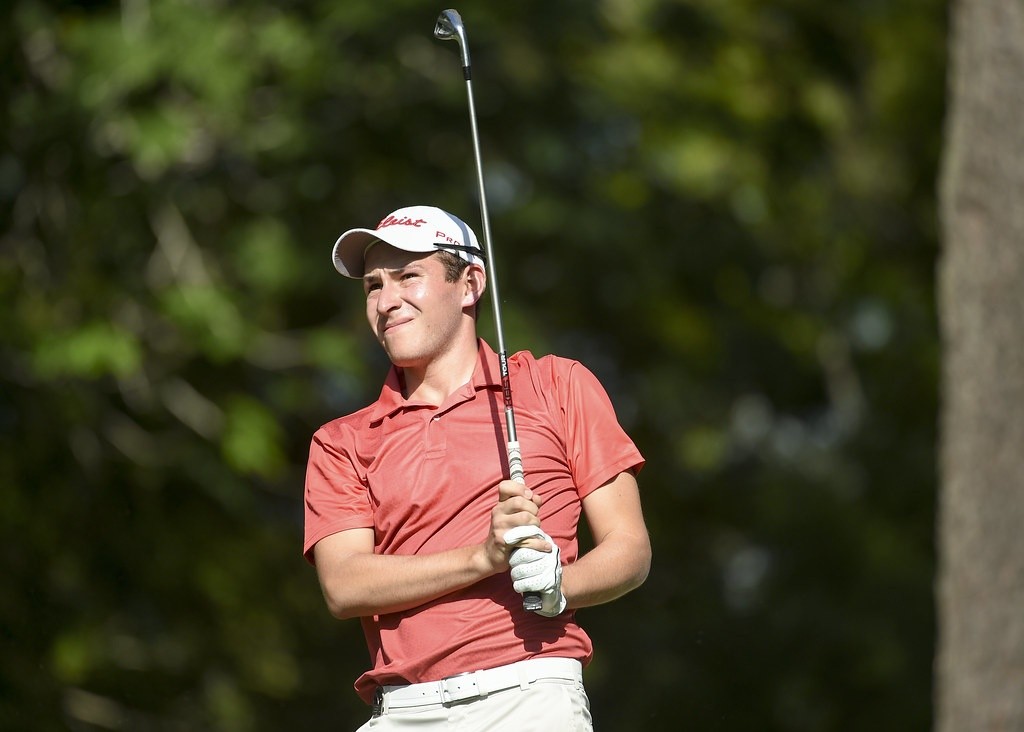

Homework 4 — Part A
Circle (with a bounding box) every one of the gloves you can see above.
[503,525,567,618]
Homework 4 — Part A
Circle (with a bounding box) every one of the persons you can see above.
[303,205,653,732]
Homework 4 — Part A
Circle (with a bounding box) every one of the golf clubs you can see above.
[433,9,541,611]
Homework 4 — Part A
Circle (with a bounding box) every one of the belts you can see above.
[378,658,584,708]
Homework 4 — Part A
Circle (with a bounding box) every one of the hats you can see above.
[332,206,485,279]
